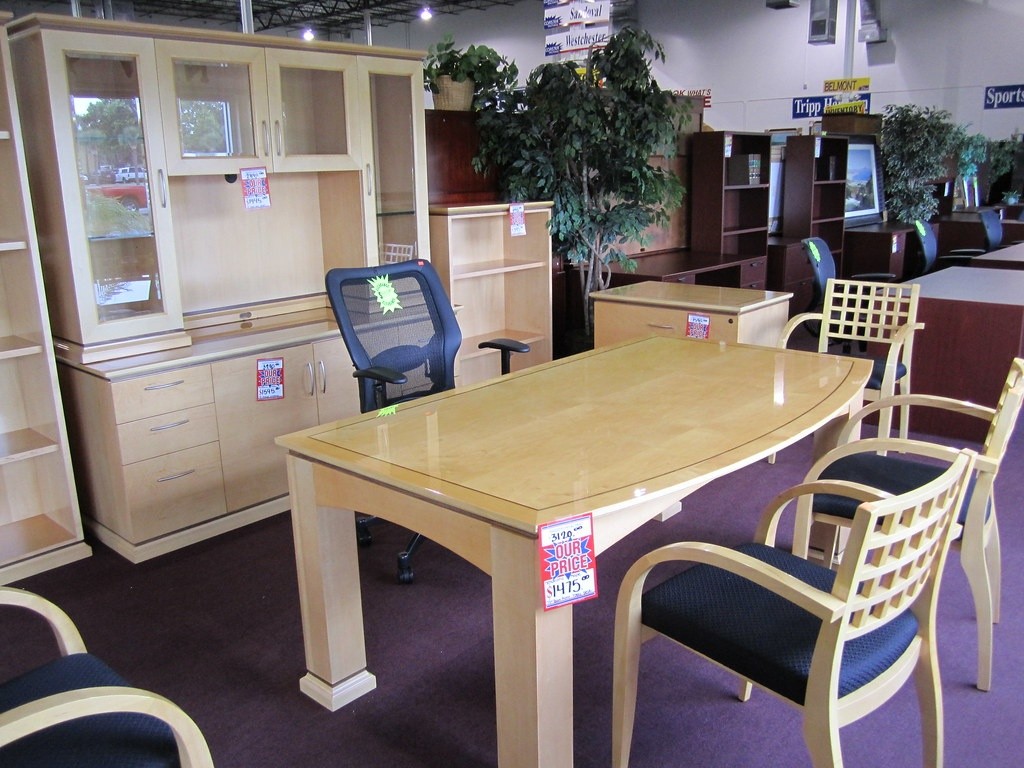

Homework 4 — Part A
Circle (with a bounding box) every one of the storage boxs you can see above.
[821,112,882,135]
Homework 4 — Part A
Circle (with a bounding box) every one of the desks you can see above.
[272,330,877,768]
[870,263,1024,444]
[968,243,1024,271]
[567,248,768,347]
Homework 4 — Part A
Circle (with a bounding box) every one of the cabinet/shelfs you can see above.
[1,10,463,565]
[688,131,776,257]
[783,135,850,296]
[0,10,93,588]
[936,212,988,267]
[766,233,813,318]
[429,201,557,388]
[588,281,795,349]
[844,220,941,282]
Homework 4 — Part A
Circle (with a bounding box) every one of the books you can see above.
[728,154,761,185]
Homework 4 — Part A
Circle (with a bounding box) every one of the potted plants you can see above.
[423,30,519,111]
[1001,190,1021,205]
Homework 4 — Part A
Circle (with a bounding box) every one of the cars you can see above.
[83,163,148,212]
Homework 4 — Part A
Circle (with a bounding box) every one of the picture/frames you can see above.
[844,135,886,229]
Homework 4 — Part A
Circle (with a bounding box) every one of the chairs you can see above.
[610,447,978,768]
[978,208,1012,253]
[0,582,214,768]
[792,352,1024,692]
[324,257,532,587]
[769,277,924,457]
[800,235,896,354]
[914,219,985,276]
